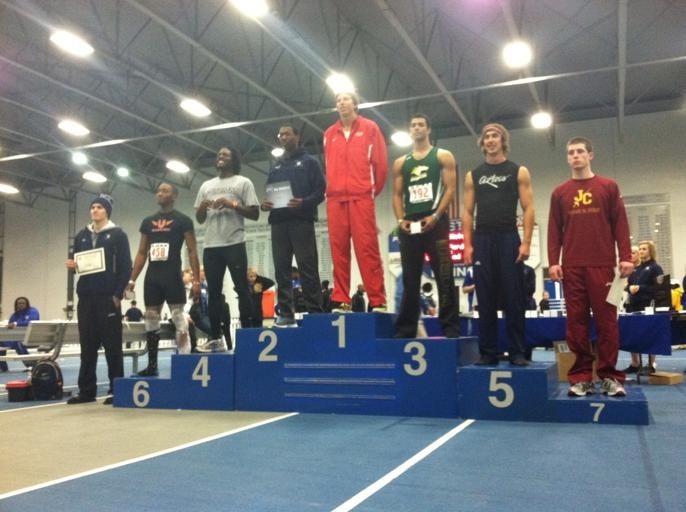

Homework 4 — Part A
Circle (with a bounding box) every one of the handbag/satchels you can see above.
[31,360,63,401]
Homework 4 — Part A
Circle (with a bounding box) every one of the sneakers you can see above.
[67,393,114,405]
[391,324,414,339]
[330,303,353,314]
[194,335,229,353]
[474,352,499,367]
[372,302,385,309]
[568,378,627,397]
[512,354,532,365]
[442,328,460,337]
[272,317,299,328]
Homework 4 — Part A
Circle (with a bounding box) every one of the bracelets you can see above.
[397,217,404,225]
[127,278,137,288]
[232,200,238,209]
[431,212,443,224]
[191,280,201,286]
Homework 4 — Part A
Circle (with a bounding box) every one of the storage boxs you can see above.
[648,371,685,388]
[3,381,34,401]
[551,336,601,383]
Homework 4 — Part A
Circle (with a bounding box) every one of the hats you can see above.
[91,196,113,216]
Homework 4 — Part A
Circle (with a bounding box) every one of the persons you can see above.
[621,241,673,372]
[275,267,372,326]
[395,259,554,351]
[390,113,461,337]
[260,122,328,328]
[191,143,259,354]
[187,266,275,353]
[0,297,39,373]
[67,193,133,404]
[462,121,536,365]
[546,137,635,399]
[121,182,202,377]
[124,300,143,348]
[324,90,388,313]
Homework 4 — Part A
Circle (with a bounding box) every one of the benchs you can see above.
[0,313,197,378]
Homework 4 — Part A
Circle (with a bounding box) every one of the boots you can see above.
[136,333,159,375]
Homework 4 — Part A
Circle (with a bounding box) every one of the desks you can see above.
[470,310,676,386]
[410,310,470,342]
